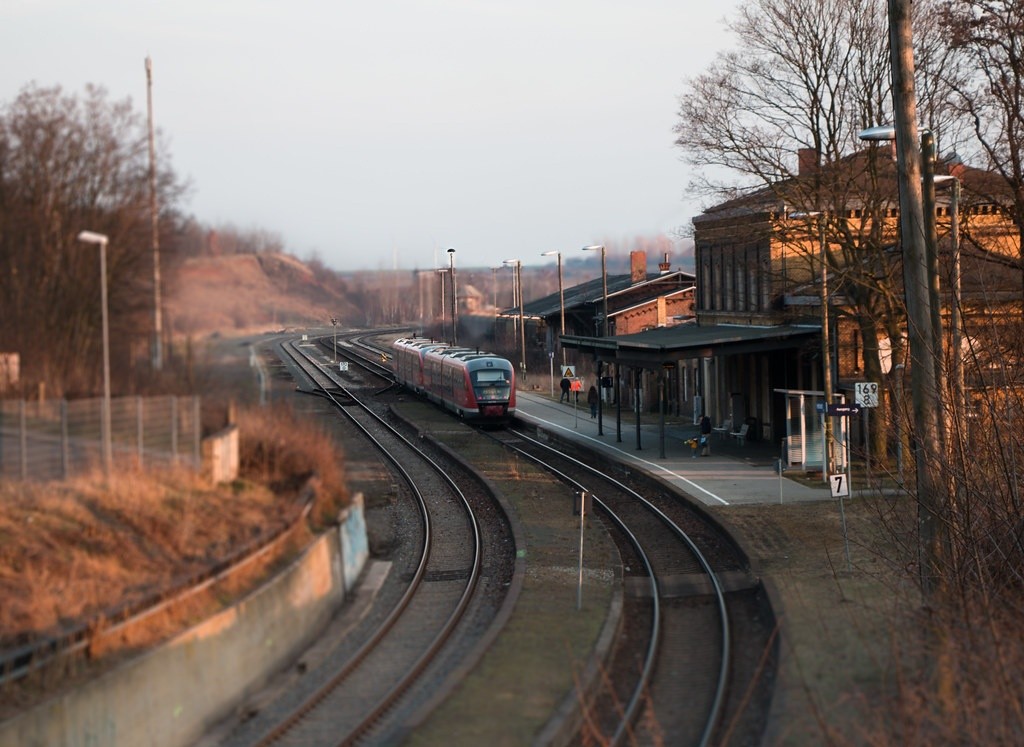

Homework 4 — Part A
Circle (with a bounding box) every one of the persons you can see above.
[688,435,698,459]
[586,385,598,418]
[571,377,584,402]
[697,414,712,457]
[559,379,571,403]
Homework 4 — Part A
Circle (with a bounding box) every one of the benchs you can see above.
[712,420,731,440]
[729,424,749,447]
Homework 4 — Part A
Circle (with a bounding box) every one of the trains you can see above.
[392,332,516,422]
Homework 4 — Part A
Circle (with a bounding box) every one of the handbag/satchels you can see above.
[700,436,708,447]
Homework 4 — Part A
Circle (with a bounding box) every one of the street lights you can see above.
[789,209,837,477]
[446,249,462,346]
[582,246,608,404]
[437,269,449,342]
[858,126,970,577]
[503,258,530,383]
[540,249,567,367]
[78,231,117,486]
[916,173,970,541]
